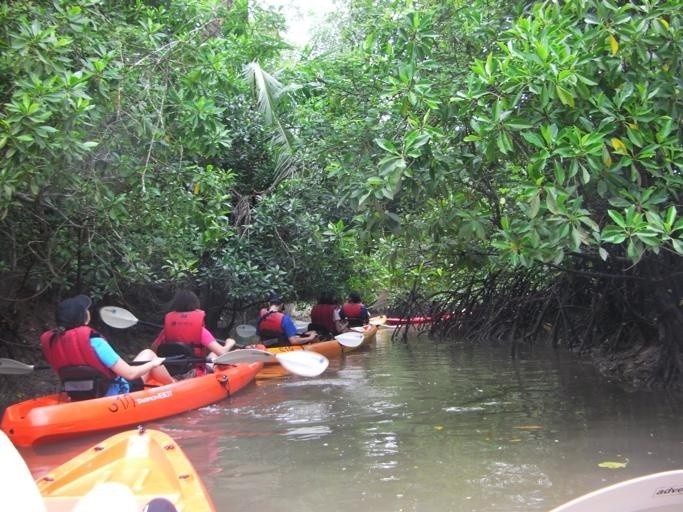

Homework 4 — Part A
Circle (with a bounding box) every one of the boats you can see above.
[2,344,267,447]
[387,313,450,325]
[35,429,217,512]
[369,315,387,326]
[234,323,376,363]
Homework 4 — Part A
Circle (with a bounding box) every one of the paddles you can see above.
[236,324,365,348]
[0,348,275,376]
[99,305,330,377]
[293,321,375,333]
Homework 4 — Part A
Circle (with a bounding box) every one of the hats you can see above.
[56,295,91,328]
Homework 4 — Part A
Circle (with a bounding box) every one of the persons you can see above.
[256,298,315,347]
[339,289,370,327]
[40,295,176,402]
[310,290,350,335]
[151,291,235,381]
